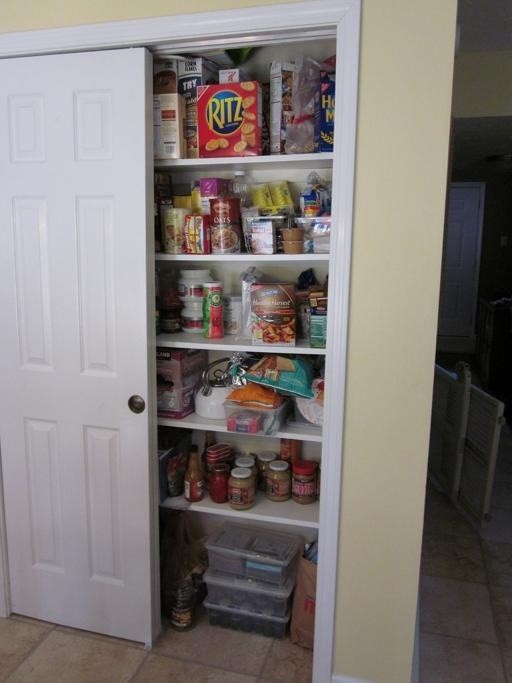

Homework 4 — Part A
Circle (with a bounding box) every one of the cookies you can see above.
[205,82,256,152]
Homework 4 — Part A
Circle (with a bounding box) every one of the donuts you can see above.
[152,70,176,94]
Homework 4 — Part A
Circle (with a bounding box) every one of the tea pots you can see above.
[196,359,241,422]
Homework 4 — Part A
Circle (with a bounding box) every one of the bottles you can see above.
[200,431,218,483]
[230,171,253,212]
[184,445,205,502]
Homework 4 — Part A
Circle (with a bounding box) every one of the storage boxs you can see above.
[289,537,318,649]
[222,393,288,437]
[157,348,209,418]
[202,521,304,639]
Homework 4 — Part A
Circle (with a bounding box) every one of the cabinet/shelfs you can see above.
[2,0,362,682]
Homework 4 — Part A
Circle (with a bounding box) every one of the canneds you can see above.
[205,443,322,511]
[163,207,192,254]
[210,197,242,255]
